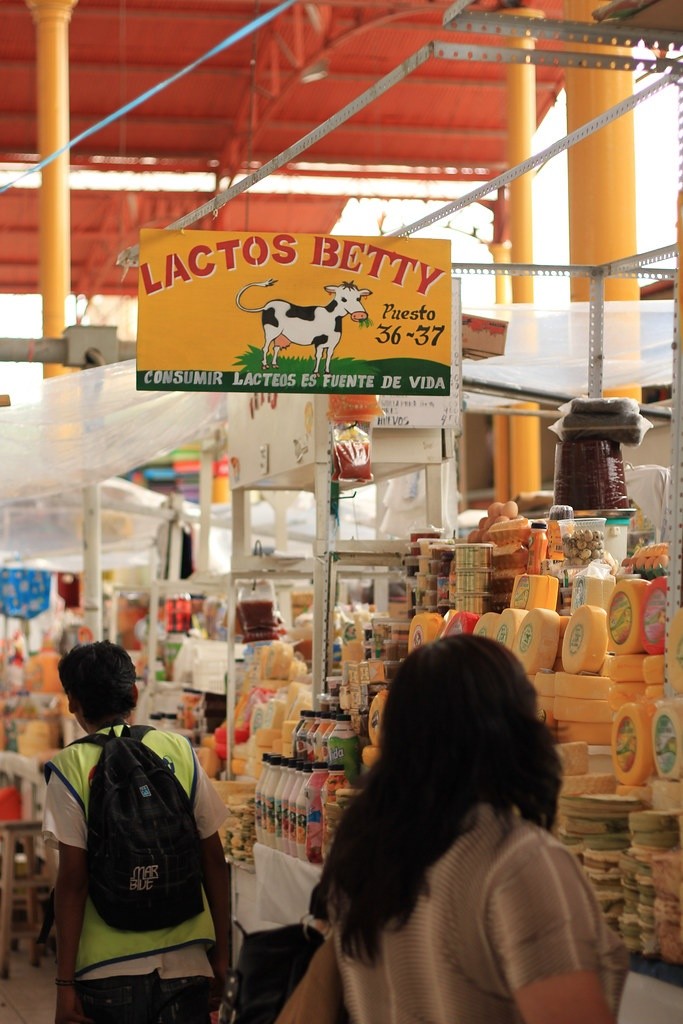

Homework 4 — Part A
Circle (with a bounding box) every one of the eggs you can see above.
[622,543,668,571]
[467,500,518,544]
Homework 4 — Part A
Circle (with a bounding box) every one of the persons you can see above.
[39,642,235,1024]
[275,632,630,1024]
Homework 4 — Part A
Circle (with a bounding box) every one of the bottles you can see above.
[256,709,360,864]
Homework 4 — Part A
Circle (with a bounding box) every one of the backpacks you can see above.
[63,717,206,933]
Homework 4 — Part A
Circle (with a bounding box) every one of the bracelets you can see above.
[55,978,76,985]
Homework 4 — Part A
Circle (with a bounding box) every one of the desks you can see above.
[252,843,323,925]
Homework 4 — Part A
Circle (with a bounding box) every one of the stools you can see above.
[0,822,59,980]
